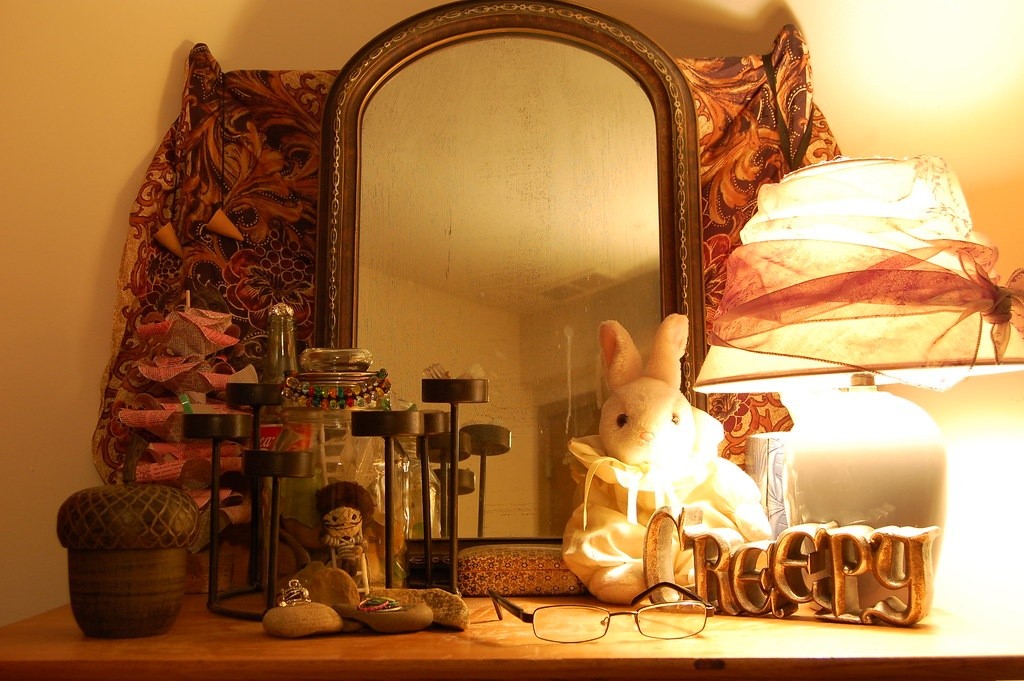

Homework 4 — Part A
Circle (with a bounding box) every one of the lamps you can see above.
[691,156,1024,614]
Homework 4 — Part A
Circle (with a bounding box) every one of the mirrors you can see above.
[314,0,703,581]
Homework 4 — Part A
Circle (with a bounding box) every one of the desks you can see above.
[0,594,1024,681]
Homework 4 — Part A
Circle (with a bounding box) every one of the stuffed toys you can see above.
[316,482,374,577]
[563,313,771,608]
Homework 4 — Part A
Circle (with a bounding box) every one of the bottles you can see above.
[260,350,407,592]
[249,304,318,592]
[393,436,441,542]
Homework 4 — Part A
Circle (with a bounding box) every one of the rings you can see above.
[277,580,311,607]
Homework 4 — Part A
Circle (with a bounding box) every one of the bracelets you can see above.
[357,596,403,611]
